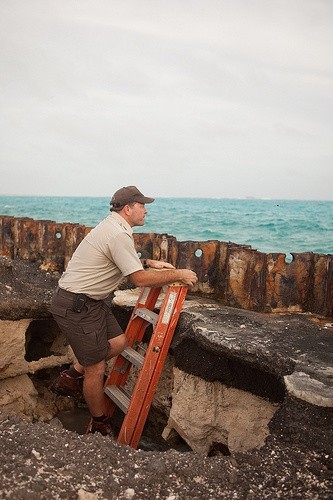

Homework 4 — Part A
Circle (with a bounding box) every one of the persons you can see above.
[48,186,199,438]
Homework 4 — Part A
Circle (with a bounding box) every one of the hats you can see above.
[109,186,154,211]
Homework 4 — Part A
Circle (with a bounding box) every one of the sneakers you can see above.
[87,414,115,437]
[50,370,86,405]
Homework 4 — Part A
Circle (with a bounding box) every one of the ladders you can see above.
[84,268,190,450]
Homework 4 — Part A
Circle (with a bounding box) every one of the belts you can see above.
[56,286,94,302]
